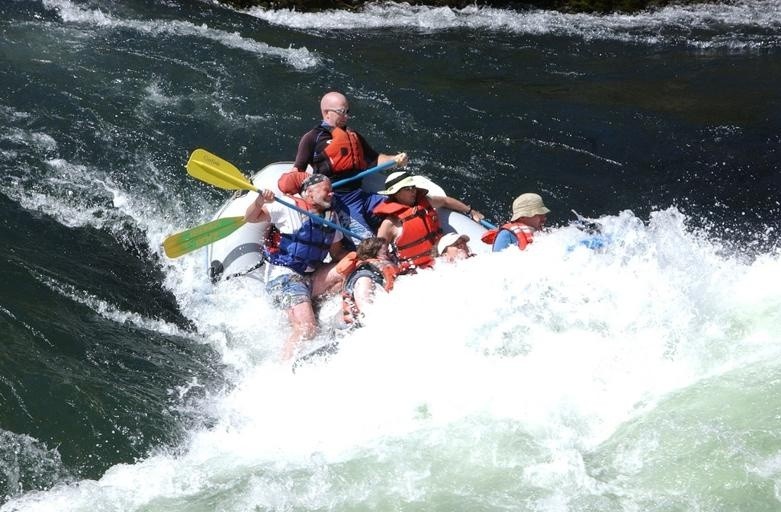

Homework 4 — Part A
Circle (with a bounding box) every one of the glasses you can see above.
[325,108,350,114]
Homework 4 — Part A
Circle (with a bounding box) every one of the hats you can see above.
[377,171,430,196]
[510,192,550,223]
[437,231,471,256]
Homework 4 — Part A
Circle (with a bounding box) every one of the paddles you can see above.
[165,157,398,258]
[187,149,403,253]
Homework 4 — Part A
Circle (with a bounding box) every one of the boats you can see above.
[204,156,496,368]
